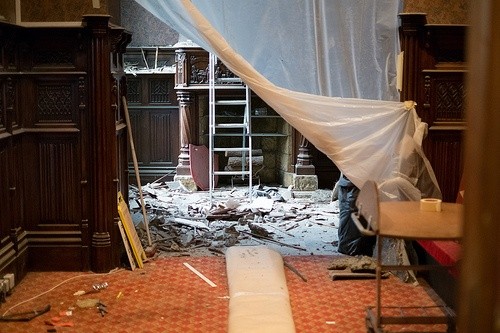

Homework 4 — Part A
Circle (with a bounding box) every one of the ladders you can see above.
[207,49,254,196]
